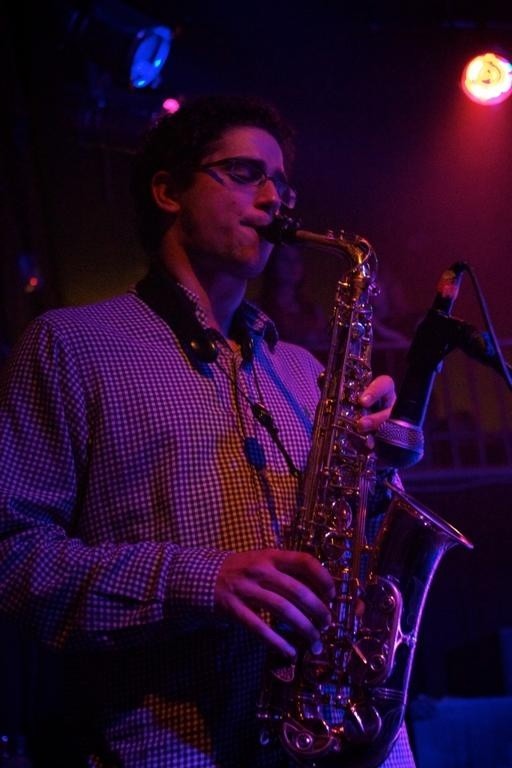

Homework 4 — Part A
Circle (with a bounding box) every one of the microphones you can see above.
[373,262,466,470]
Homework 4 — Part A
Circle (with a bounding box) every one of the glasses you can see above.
[181,156,297,211]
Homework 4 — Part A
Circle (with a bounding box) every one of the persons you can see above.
[252,232,329,350]
[1,94,419,767]
[358,274,419,358]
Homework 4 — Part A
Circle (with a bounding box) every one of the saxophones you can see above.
[249,213,475,767]
